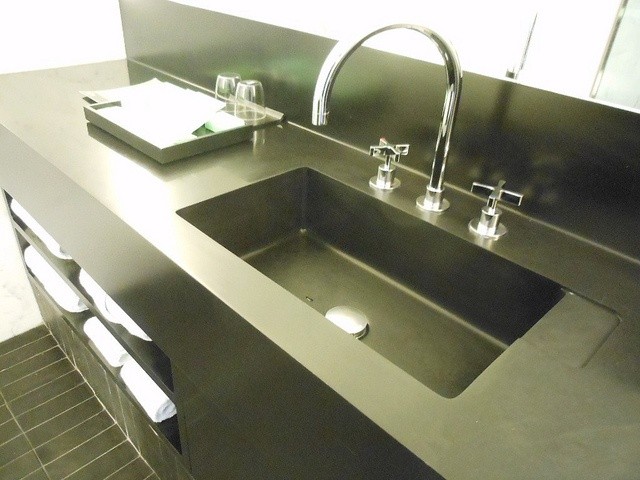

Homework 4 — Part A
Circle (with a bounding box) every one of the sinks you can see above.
[176,166,565,398]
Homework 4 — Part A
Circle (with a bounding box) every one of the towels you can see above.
[22,243,87,314]
[106,295,153,343]
[10,199,73,260]
[78,269,107,321]
[82,316,126,368]
[119,359,177,422]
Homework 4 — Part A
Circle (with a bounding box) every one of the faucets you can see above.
[310,22,463,213]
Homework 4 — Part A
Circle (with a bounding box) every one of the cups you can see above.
[216,74,240,101]
[235,79,267,121]
